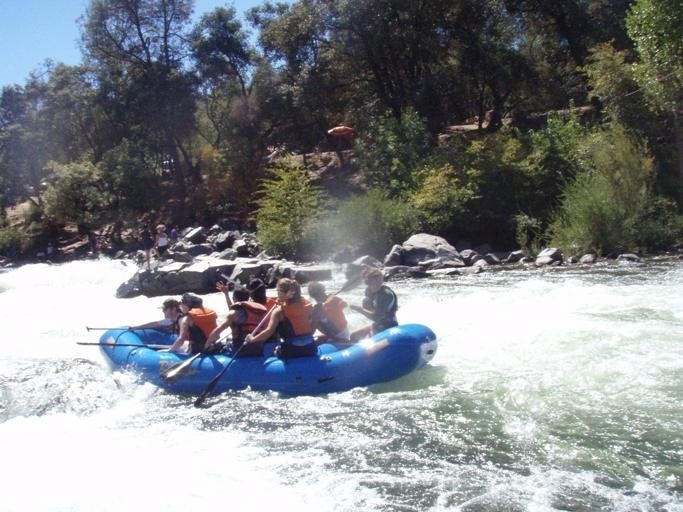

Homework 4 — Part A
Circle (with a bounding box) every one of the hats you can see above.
[157,300,179,309]
[182,292,202,307]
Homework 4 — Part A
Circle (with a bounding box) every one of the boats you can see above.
[98,321,439,400]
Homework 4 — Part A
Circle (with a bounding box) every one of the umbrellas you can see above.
[327,124,353,150]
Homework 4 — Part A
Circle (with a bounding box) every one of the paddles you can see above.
[194,304,277,407]
[159,332,233,382]
[334,275,361,296]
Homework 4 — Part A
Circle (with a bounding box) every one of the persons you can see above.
[246,279,276,311]
[349,267,399,343]
[170,225,180,245]
[307,282,351,346]
[128,300,190,354]
[152,223,171,259]
[360,267,375,312]
[202,279,269,358]
[155,292,223,355]
[241,278,319,359]
